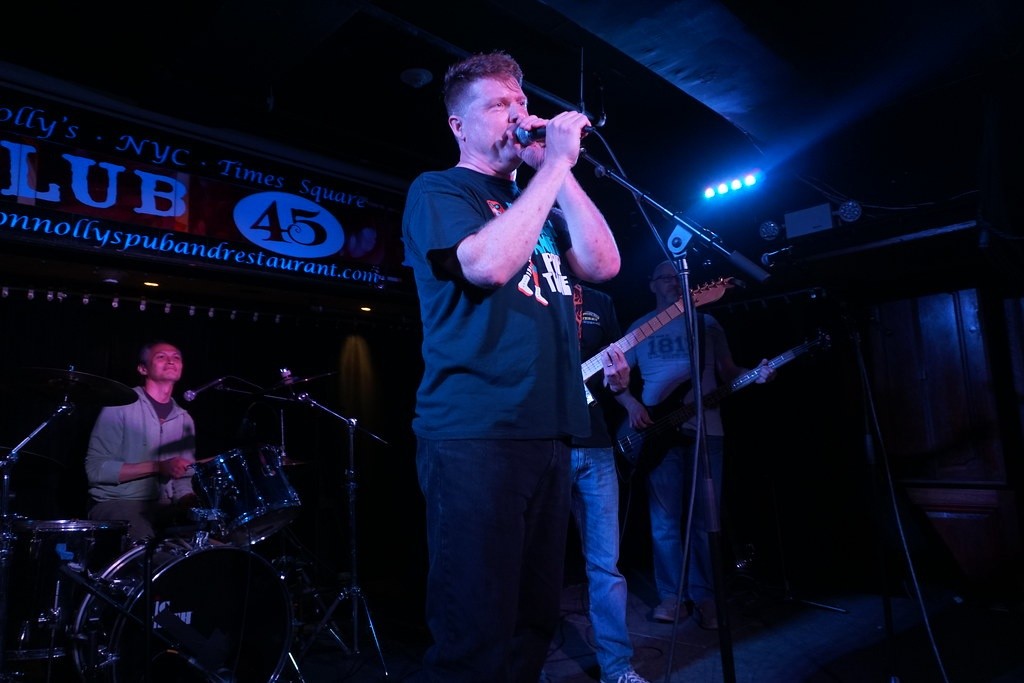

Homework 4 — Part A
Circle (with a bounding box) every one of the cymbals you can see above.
[244,368,332,396]
[5,363,140,407]
[0,446,64,479]
[280,453,304,466]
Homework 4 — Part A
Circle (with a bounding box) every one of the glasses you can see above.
[652,274,683,284]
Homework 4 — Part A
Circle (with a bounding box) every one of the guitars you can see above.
[581,276,747,410]
[613,326,834,474]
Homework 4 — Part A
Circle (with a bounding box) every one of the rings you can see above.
[608,364,612,367]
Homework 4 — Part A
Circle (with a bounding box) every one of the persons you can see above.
[85,343,195,553]
[615,260,777,628]
[569,282,648,683]
[401,53,621,683]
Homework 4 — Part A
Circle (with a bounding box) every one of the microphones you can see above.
[184,378,224,401]
[762,246,796,268]
[514,126,596,147]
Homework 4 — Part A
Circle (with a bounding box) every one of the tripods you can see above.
[290,386,393,679]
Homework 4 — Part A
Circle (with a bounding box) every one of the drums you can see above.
[71,531,295,683]
[13,519,132,565]
[188,444,302,547]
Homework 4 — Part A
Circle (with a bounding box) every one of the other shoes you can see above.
[653,598,690,621]
[600,668,650,683]
[693,600,718,629]
